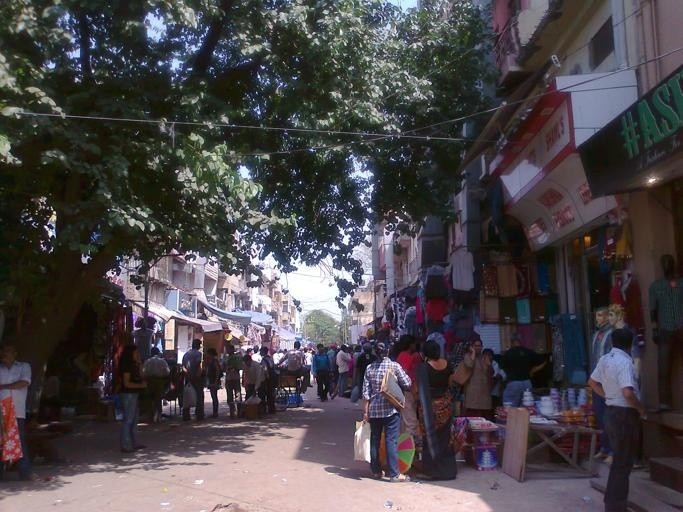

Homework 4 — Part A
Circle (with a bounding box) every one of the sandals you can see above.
[390,472,410,482]
[373,470,385,479]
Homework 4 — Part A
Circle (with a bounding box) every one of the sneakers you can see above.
[27,472,41,481]
[122,444,145,451]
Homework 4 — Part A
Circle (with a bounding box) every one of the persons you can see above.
[0,335,41,483]
[362,342,411,480]
[312,337,379,401]
[120,345,169,451]
[589,308,615,465]
[585,328,649,512]
[649,254,682,414]
[222,341,312,418]
[182,338,221,420]
[396,334,547,477]
[616,267,646,348]
[605,302,645,469]
[600,207,618,272]
[613,207,635,272]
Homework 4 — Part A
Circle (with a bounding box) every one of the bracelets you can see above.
[651,321,657,328]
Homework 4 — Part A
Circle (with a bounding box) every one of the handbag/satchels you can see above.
[380,362,406,409]
[451,361,472,384]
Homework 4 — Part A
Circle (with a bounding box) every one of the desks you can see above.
[495,405,603,483]
